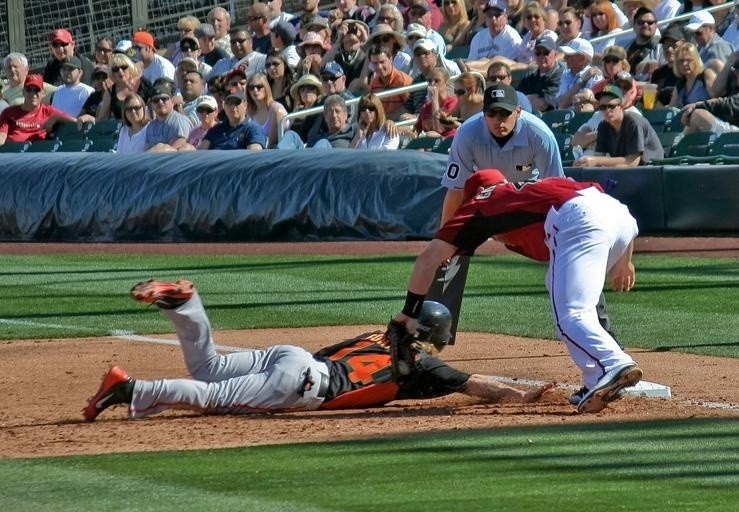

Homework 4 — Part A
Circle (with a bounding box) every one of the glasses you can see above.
[488,74,508,82]
[125,105,140,111]
[361,105,375,113]
[264,62,280,69]
[535,50,550,55]
[454,89,466,96]
[249,84,264,91]
[598,104,620,112]
[486,109,512,119]
[247,16,261,21]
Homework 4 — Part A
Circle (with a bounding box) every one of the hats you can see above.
[271,1,436,100]
[152,23,247,109]
[659,29,685,42]
[483,0,508,13]
[595,45,633,99]
[23,29,157,91]
[534,36,555,51]
[484,83,516,113]
[560,38,594,56]
[685,11,716,29]
[460,167,510,202]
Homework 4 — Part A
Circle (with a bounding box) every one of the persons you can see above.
[84,280,558,421]
[380,168,640,413]
[424,81,623,352]
[0,0,738,172]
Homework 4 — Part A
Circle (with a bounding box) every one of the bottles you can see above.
[572,145,585,160]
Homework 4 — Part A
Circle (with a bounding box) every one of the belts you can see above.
[554,191,581,211]
[317,373,331,398]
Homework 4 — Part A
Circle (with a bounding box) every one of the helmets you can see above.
[415,301,453,344]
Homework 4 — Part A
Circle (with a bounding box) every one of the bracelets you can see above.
[402,290,426,319]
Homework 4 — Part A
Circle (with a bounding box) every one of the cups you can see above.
[640,83,659,109]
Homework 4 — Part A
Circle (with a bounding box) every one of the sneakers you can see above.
[130,279,192,308]
[569,364,642,412]
[81,366,132,421]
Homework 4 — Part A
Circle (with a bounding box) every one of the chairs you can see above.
[404,44,739,165]
[0,117,124,153]
